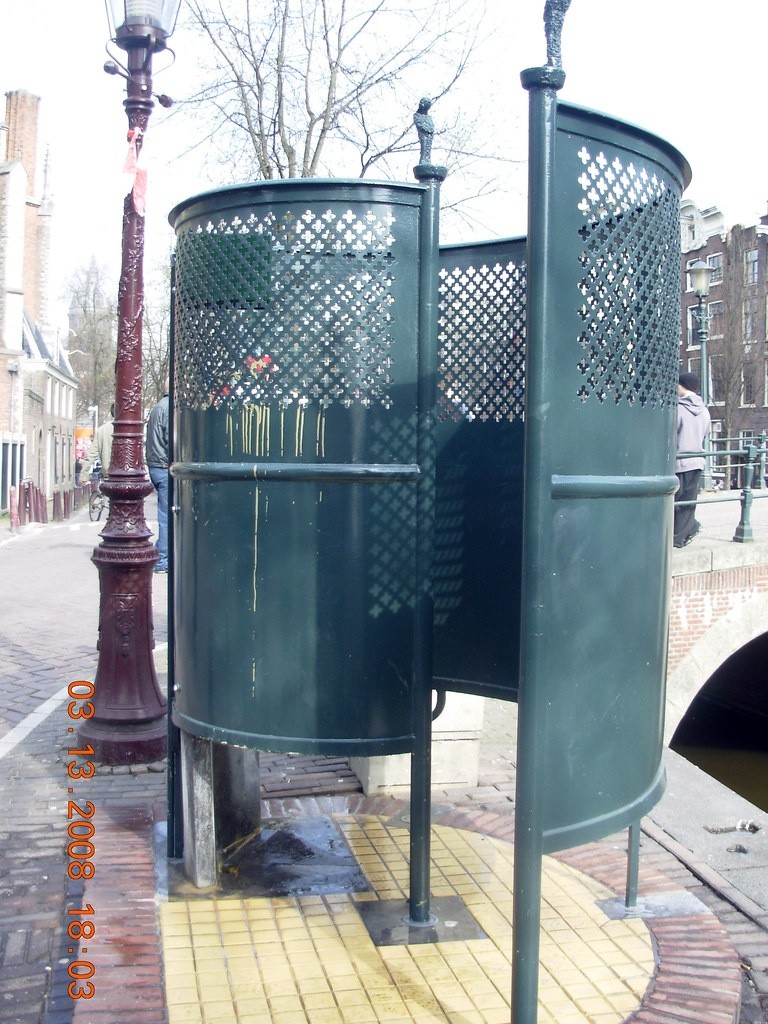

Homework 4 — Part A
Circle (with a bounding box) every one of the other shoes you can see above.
[684,522,702,546]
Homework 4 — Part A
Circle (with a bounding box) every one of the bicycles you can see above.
[89,486,110,521]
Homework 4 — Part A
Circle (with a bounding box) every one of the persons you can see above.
[674,373,711,548]
[79,403,116,486]
[75,459,81,485]
[147,376,170,572]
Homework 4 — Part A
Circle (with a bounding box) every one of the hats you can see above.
[678,372,700,392]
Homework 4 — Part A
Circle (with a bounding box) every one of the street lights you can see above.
[681,257,717,492]
[74,1,187,768]
